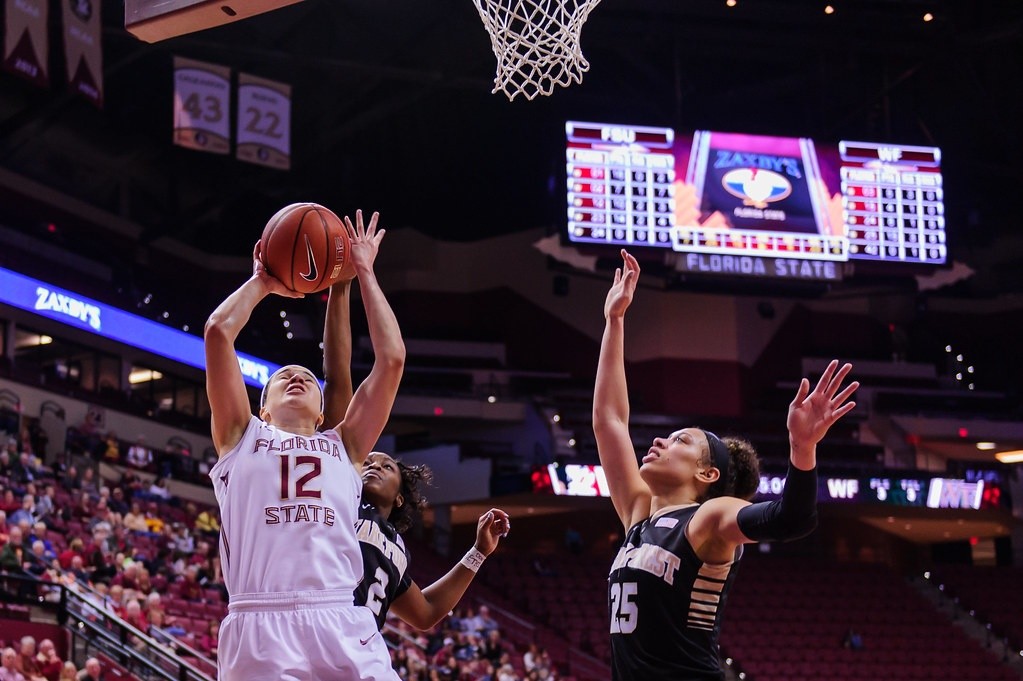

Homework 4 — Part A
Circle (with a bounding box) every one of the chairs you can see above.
[2,381,1022,681]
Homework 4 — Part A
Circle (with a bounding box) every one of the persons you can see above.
[0,388,230,680]
[203,209,408,681]
[593,250,860,681]
[374,596,581,681]
[320,220,510,638]
[837,627,869,657]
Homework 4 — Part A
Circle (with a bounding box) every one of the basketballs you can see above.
[260,202,351,292]
